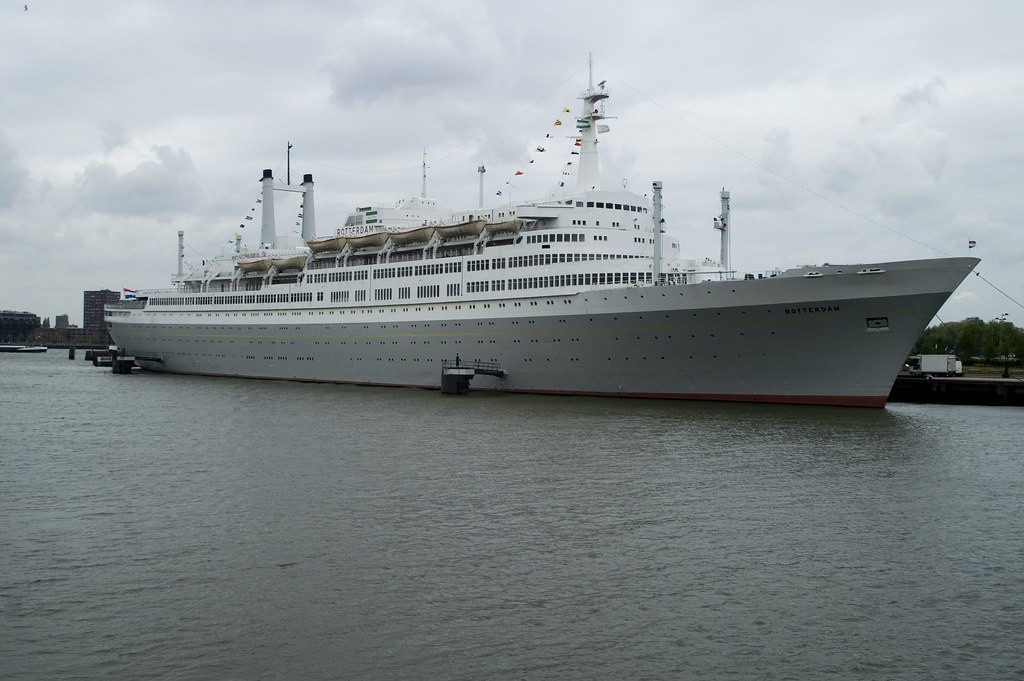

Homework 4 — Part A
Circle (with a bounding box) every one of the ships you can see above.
[101,52,984,418]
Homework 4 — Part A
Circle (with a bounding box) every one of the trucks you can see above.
[910,355,956,377]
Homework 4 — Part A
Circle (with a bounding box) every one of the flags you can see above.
[969,241,976,248]
[293,205,303,234]
[496,108,582,197]
[228,198,262,243]
[124,289,135,299]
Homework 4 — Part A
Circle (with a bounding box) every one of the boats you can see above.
[345,229,391,250]
[485,219,526,234]
[305,234,348,254]
[0,334,48,353]
[432,218,487,240]
[269,255,307,270]
[387,222,436,246]
[234,256,275,272]
